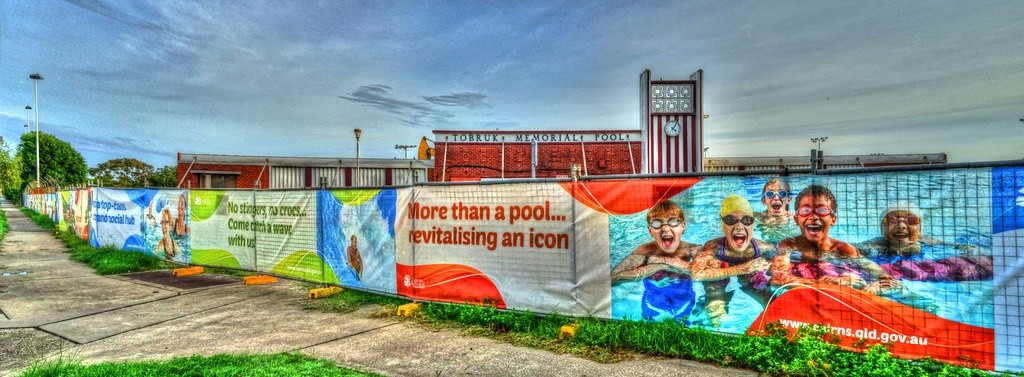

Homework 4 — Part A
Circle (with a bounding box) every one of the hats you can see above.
[880,195,924,226]
[720,194,752,228]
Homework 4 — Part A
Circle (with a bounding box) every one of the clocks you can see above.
[664,120,681,137]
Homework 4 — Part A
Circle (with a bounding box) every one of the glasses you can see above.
[763,189,788,199]
[796,203,835,216]
[648,217,684,230]
[720,212,755,226]
[882,212,921,227]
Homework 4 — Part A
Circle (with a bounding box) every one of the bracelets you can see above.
[954,244,960,249]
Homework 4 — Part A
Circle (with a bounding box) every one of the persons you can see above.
[605,176,987,326]
[30,196,190,262]
[347,235,363,280]
